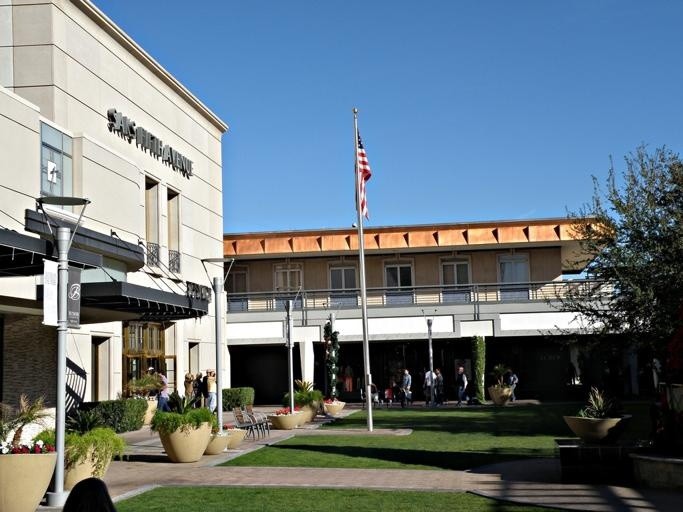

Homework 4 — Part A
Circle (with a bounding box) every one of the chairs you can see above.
[360,383,411,409]
[232,404,271,441]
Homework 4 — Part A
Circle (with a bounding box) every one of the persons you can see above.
[507,369,518,402]
[156,368,172,413]
[399,369,412,408]
[455,367,468,406]
[184,369,217,412]
[424,366,443,408]
[62,477,117,512]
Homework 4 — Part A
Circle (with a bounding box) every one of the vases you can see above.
[0,452,56,512]
[226,429,246,448]
[269,411,304,429]
[325,402,345,415]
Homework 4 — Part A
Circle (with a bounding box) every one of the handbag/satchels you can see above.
[405,390,412,400]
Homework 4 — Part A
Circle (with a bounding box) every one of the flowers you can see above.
[324,399,332,404]
[274,406,298,414]
[0,444,54,454]
[222,423,234,429]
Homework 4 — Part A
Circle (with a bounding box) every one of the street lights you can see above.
[323,302,343,397]
[36,196,91,506]
[422,307,439,408]
[277,286,302,414]
[201,257,235,434]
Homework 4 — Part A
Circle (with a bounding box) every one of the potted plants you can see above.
[284,379,325,422]
[203,414,232,454]
[125,371,166,424]
[563,385,632,445]
[488,363,515,405]
[149,391,219,462]
[32,410,129,491]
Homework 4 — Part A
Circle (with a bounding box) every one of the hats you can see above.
[206,369,212,373]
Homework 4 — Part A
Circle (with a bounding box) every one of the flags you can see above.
[354,130,372,221]
